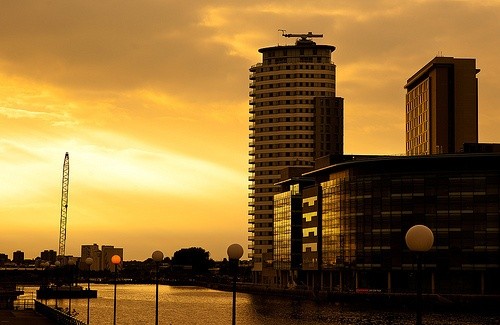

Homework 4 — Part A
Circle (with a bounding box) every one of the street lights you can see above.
[55,261,61,309]
[227,244,244,325]
[86,257,93,325]
[44,262,50,305]
[111,255,121,325]
[405,224,434,324]
[67,259,74,315]
[40,262,45,303]
[152,251,165,325]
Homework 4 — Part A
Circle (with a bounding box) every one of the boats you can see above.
[37,280,97,298]
